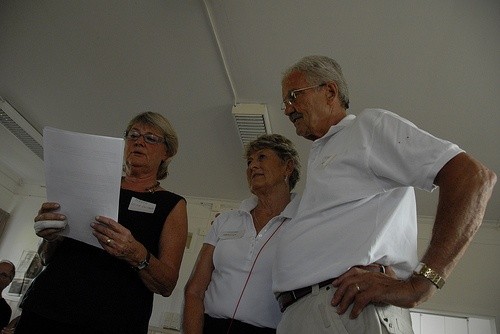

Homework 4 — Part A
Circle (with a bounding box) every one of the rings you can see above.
[356,283,360,293]
[107,237,112,246]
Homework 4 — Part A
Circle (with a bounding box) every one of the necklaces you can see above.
[254,208,282,230]
[121,177,160,193]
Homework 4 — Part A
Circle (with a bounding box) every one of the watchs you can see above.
[131,250,151,271]
[413,262,445,290]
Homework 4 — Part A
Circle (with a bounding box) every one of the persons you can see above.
[0,259,15,334]
[182,134,302,334]
[210,56,497,334]
[15,111,189,334]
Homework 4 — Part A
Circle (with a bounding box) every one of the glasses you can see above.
[278,83,323,112]
[122,128,168,146]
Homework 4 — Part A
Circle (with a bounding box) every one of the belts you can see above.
[273,277,338,312]
